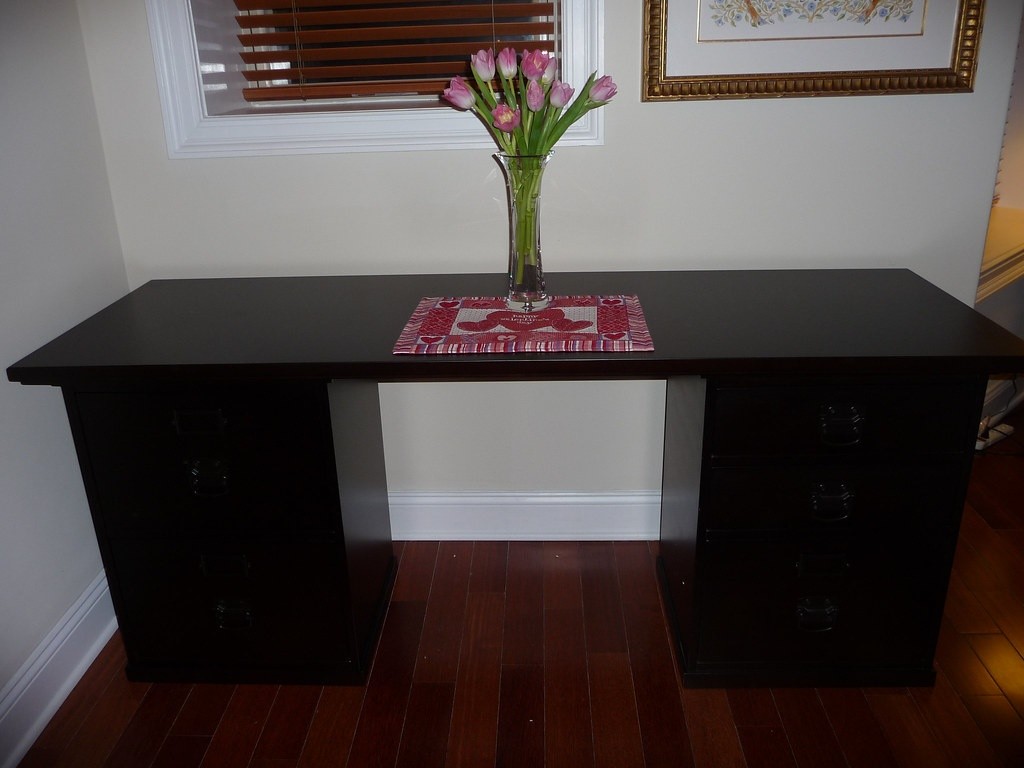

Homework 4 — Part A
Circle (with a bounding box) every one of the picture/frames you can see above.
[641,0,986,102]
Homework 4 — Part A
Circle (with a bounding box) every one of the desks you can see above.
[4,267,1024,689]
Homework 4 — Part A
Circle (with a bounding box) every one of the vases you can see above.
[494,149,555,302]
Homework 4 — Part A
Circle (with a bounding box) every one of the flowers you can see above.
[440,46,619,155]
